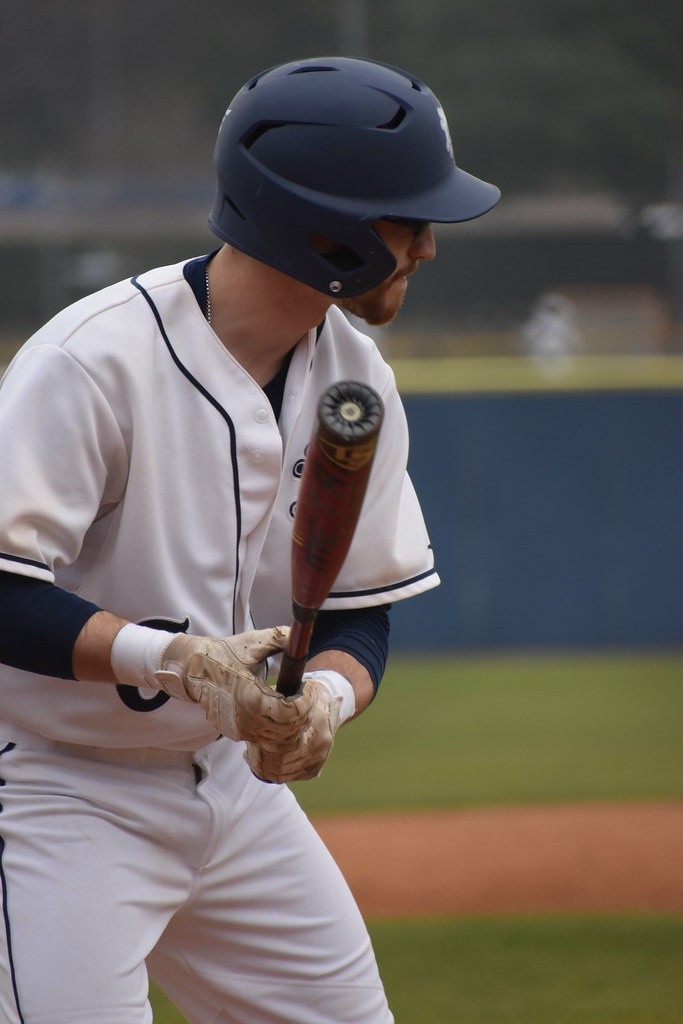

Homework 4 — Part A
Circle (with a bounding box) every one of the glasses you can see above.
[379,216,429,244]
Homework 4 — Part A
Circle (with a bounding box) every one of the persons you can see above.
[0,57,503,1024]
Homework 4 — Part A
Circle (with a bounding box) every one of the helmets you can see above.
[207,57,501,298]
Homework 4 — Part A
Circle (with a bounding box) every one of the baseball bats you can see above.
[278,380,385,702]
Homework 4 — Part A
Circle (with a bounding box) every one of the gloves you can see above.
[110,625,315,753]
[243,670,356,784]
